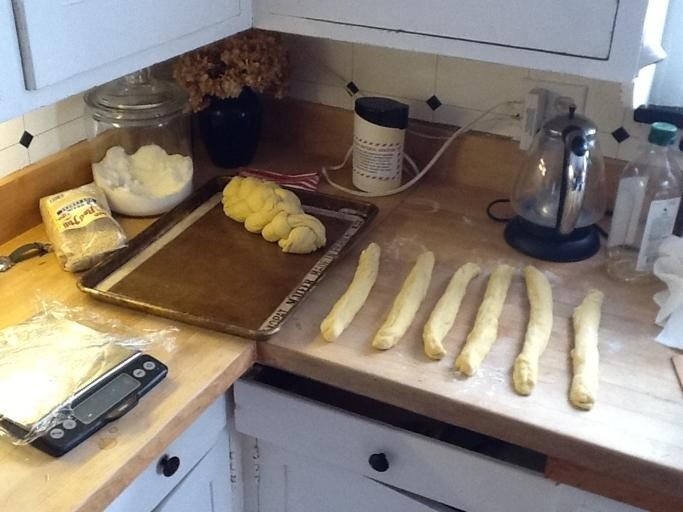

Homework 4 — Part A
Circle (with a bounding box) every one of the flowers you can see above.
[172,29,296,113]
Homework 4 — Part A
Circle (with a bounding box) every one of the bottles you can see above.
[350,95,410,195]
[82,65,195,220]
[602,122,683,287]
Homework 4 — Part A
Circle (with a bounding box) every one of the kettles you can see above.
[503,101,611,264]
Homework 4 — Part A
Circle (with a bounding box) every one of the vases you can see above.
[196,86,264,169]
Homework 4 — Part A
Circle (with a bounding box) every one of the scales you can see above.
[0,309,168,457]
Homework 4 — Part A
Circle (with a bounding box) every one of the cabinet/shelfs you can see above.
[232,366,683,512]
[252,1,669,112]
[97,385,244,512]
[1,0,252,125]
